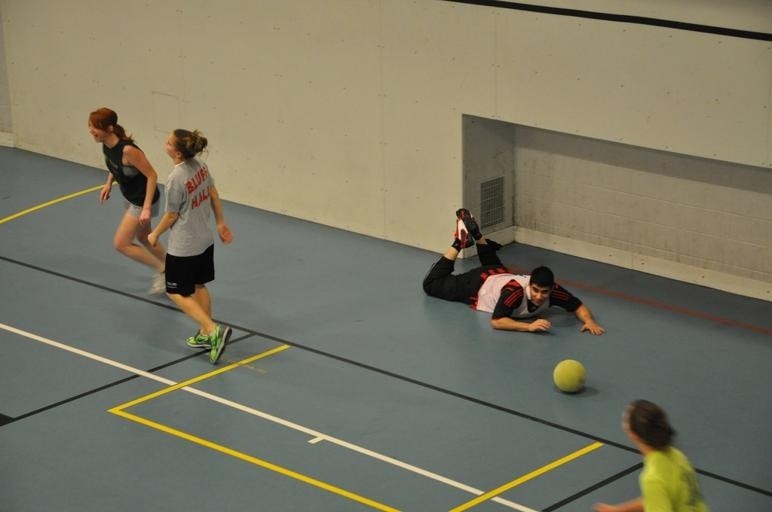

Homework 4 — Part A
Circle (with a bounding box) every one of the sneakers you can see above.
[186,330,211,348]
[209,325,232,364]
[454,208,480,249]
[150,272,166,296]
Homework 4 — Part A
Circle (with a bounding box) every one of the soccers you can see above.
[554,359,586,393]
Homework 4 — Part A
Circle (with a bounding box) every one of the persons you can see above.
[594,401,711,512]
[147,128,234,366]
[88,108,169,295]
[422,208,605,336]
